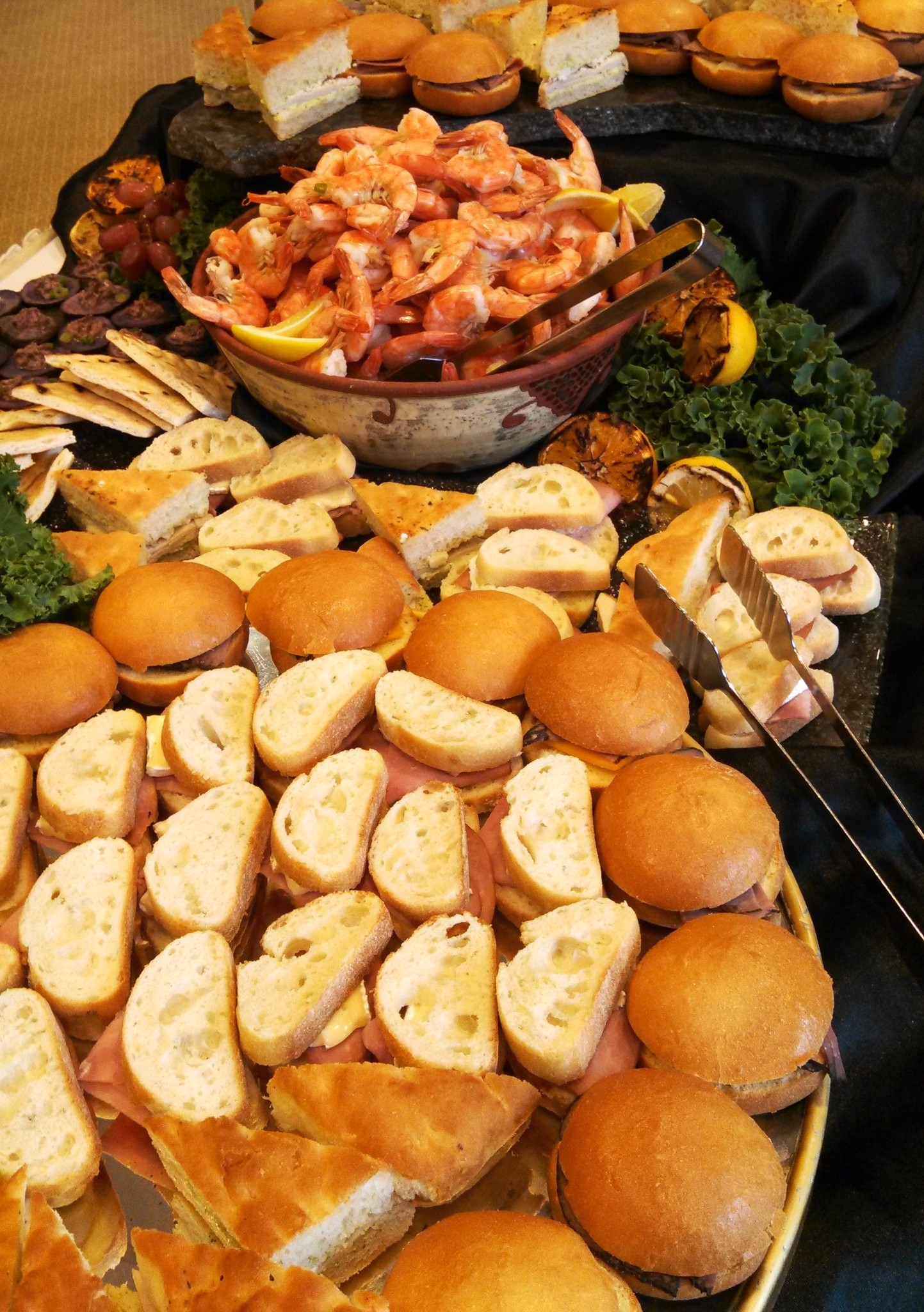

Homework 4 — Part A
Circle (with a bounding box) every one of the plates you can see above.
[0,730,833,1312]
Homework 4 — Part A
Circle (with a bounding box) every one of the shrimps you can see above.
[160,108,644,382]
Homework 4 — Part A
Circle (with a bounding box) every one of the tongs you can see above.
[404,212,720,392]
[632,506,924,957]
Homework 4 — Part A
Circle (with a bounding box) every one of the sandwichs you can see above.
[244,24,361,141]
[191,5,262,112]
[384,0,629,110]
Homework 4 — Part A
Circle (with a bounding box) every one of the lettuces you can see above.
[605,217,908,520]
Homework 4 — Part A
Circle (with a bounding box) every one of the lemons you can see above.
[230,291,329,363]
[546,181,666,237]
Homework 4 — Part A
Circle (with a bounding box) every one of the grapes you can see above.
[98,179,190,275]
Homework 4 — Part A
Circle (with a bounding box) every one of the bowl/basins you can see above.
[192,173,661,473]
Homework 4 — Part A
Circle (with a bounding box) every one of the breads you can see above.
[1,329,883,746]
[0,650,642,1312]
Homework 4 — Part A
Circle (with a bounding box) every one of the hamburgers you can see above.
[626,913,849,1119]
[248,1,523,118]
[544,1069,787,1301]
[614,0,924,123]
[598,754,789,932]
[522,631,693,795]
